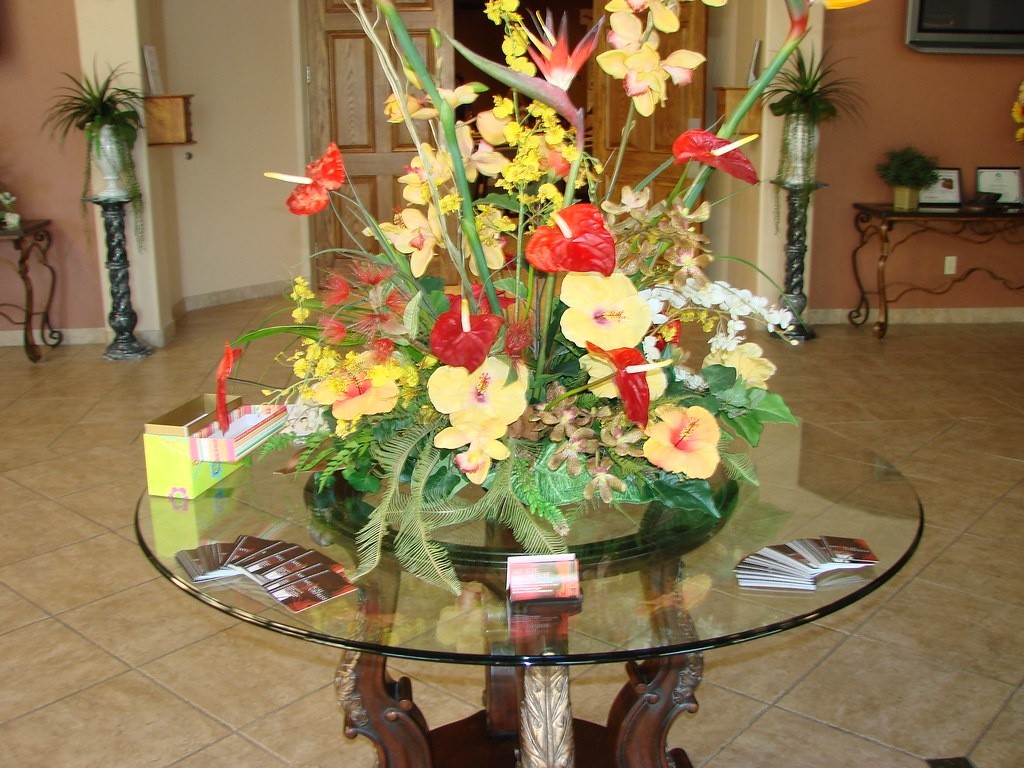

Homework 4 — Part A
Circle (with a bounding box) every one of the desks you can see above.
[129,395,929,768]
[0,217,66,365]
[849,201,1024,339]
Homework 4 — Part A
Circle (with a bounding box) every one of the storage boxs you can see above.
[141,394,290,502]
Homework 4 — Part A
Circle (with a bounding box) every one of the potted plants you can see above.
[873,146,942,211]
[39,51,167,254]
[758,45,870,245]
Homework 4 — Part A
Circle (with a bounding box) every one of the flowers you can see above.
[210,0,879,595]
[1010,81,1023,143]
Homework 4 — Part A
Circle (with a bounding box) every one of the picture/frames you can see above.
[916,167,963,208]
[976,166,1021,206]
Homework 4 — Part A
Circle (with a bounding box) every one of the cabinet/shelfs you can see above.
[143,93,197,145]
[713,84,759,135]
